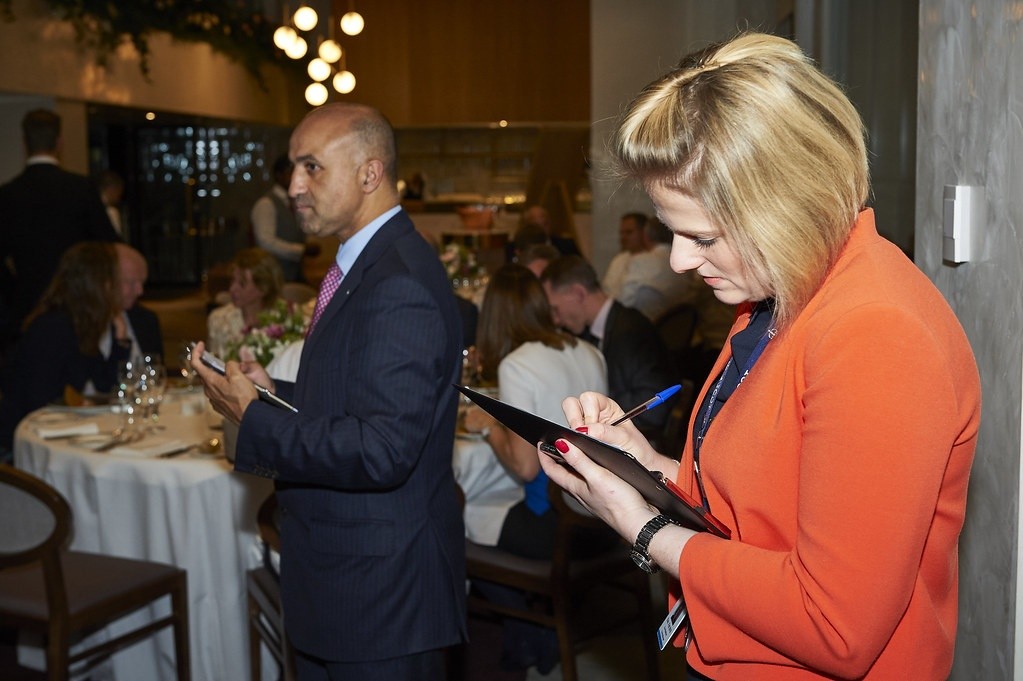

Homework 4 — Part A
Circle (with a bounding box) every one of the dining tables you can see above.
[15,378,521,681]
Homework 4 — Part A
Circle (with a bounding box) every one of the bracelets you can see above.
[482,420,503,440]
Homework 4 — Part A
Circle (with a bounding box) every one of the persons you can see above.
[453,213,739,559]
[1,108,167,449]
[248,150,323,298]
[190,102,472,681]
[538,32,983,681]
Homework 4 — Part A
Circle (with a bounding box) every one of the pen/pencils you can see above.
[612,384,681,427]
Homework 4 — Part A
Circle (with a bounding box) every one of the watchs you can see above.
[629,515,682,577]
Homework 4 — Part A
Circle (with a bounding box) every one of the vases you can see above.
[453,263,474,280]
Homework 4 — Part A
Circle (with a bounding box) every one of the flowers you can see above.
[441,244,479,279]
[220,299,311,369]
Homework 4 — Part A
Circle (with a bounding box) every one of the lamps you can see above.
[273,0,365,106]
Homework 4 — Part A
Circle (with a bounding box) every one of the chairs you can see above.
[652,302,699,354]
[246,481,298,681]
[465,471,662,681]
[641,379,694,459]
[0,463,192,681]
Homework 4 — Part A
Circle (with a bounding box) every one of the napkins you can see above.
[146,439,190,457]
[37,422,98,439]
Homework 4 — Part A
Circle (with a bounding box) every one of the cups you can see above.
[109,350,208,443]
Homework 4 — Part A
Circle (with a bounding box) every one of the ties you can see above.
[112,312,126,340]
[305,264,344,341]
